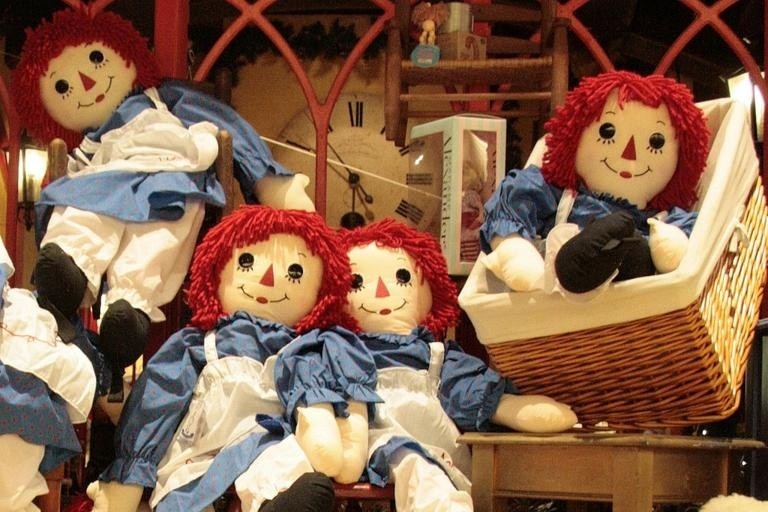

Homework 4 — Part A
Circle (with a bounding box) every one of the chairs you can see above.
[384,0,569,148]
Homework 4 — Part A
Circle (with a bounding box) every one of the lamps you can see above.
[3,127,49,232]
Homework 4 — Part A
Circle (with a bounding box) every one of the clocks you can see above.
[215,0,525,291]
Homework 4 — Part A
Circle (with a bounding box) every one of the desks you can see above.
[34,419,97,512]
[455,429,767,511]
[222,480,398,511]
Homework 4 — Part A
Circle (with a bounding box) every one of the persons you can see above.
[325,223,580,511]
[0,230,104,512]
[6,9,316,364]
[478,69,712,298]
[78,200,343,512]
